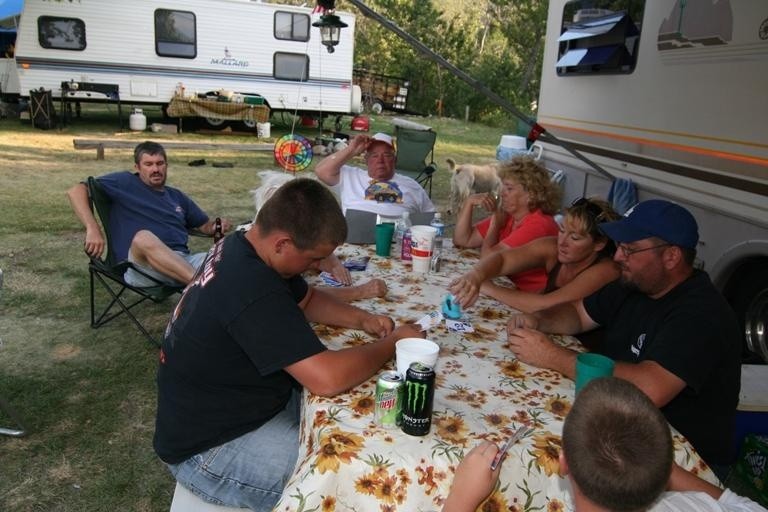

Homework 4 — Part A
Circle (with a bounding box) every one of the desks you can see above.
[172,96,271,136]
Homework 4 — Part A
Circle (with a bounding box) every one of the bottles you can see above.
[395,212,445,265]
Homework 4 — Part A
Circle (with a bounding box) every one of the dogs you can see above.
[446,158,504,221]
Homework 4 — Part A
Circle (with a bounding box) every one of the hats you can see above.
[600,200,698,247]
[371,132,394,146]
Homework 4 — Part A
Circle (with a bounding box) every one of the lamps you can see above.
[312,0,349,53]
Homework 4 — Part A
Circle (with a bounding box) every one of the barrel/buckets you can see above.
[257,122,271,139]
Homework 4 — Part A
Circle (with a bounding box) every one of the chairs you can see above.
[85,175,208,352]
[394,125,437,200]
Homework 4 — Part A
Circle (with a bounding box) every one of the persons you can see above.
[505,198,743,487]
[153,178,430,512]
[251,167,388,302]
[451,153,563,293]
[67,141,230,286]
[445,195,624,350]
[314,131,436,238]
[441,376,767,512]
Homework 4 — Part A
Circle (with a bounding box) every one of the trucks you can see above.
[535,0,767,385]
[0,0,364,133]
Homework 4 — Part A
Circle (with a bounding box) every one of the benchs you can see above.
[73,134,285,160]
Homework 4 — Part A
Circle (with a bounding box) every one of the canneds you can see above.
[400,362,437,436]
[374,370,404,432]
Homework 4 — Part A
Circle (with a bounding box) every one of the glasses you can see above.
[572,195,606,223]
[616,243,672,257]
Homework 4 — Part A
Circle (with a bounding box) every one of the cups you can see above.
[396,337,441,374]
[375,224,393,257]
[574,353,615,392]
[411,224,435,275]
